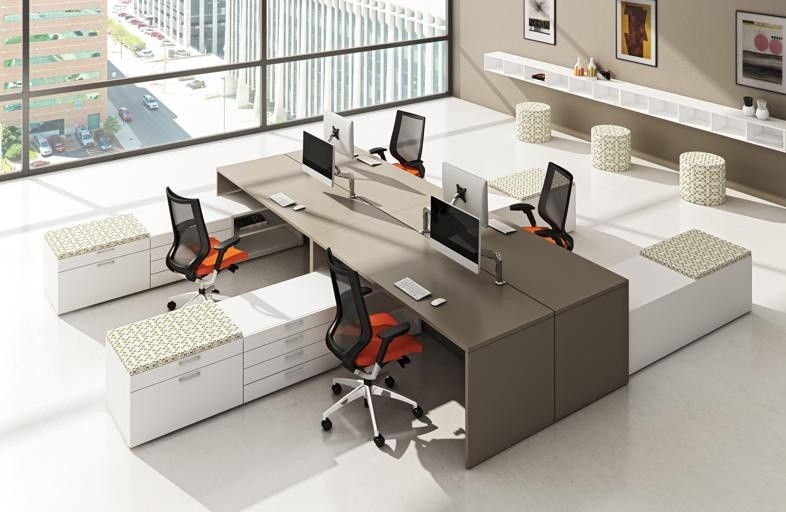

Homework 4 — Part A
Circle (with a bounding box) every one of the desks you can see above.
[285,144,629,422]
[217,153,554,470]
[483,50,786,154]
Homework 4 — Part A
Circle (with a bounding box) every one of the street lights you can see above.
[219,77,226,133]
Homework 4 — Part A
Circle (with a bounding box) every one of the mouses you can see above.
[430,297,447,307]
[354,154,358,157]
[293,205,306,211]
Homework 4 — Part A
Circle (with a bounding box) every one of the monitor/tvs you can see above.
[323,109,354,160]
[302,130,335,187]
[428,195,481,276]
[441,162,488,230]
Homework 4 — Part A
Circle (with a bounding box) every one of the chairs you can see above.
[165,186,248,309]
[510,159,575,253]
[369,110,426,179]
[321,248,424,447]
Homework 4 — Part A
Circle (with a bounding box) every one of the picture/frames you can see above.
[735,10,786,95]
[523,0,556,45]
[615,0,657,67]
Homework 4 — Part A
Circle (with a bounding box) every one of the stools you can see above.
[678,151,726,206]
[591,125,632,172]
[515,102,552,143]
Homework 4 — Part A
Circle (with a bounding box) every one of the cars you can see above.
[118,106,132,122]
[143,95,159,109]
[112,1,204,89]
[96,129,112,150]
[51,134,64,153]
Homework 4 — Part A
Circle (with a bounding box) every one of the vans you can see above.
[33,134,51,156]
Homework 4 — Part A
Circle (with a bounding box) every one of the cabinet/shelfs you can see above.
[105,265,423,450]
[487,168,577,234]
[43,192,305,316]
[608,228,753,378]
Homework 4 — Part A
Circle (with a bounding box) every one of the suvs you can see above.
[75,126,94,147]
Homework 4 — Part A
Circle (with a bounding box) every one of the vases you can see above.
[573,55,598,78]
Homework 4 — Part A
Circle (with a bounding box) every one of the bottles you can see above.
[755,100,769,120]
[574,56,585,76]
[586,57,597,77]
[742,96,754,117]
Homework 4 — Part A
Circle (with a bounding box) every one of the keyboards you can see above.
[393,276,432,301]
[356,156,382,167]
[269,192,296,208]
[488,219,517,235]
